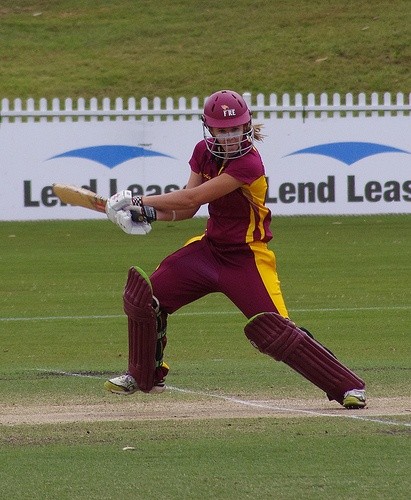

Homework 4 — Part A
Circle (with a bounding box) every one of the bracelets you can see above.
[171,210,176,221]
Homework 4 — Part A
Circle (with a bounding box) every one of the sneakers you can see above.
[104,373,166,396]
[344,388,368,408]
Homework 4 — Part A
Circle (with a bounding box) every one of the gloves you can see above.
[105,190,157,235]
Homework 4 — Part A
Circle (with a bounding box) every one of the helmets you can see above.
[201,90,255,159]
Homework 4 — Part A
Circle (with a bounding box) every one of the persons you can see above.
[103,89,369,410]
